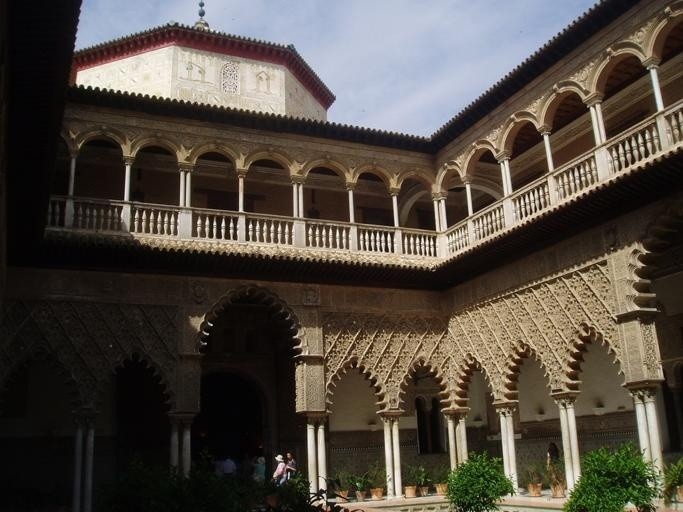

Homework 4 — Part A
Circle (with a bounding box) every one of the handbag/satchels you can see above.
[252,473,259,482]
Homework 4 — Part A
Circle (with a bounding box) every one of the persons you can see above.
[250,456,266,485]
[273,454,288,485]
[285,451,297,478]
[547,442,560,496]
[221,456,236,477]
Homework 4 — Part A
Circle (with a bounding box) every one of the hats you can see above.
[257,456,266,464]
[274,455,285,462]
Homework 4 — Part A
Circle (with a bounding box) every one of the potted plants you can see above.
[662,458,683,506]
[525,464,566,498]
[327,472,451,502]
[264,480,281,507]
